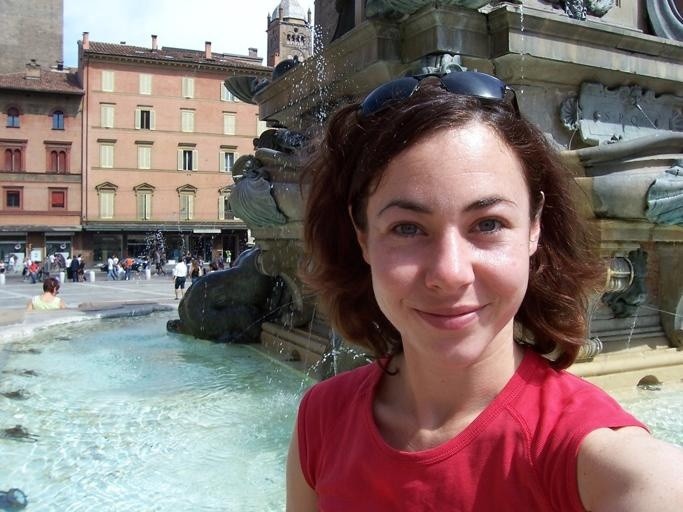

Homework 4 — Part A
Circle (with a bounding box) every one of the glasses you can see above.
[355,70,522,118]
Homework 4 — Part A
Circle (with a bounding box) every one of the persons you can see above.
[285,75,683,512]
[144,250,167,276]
[107,253,132,280]
[23,253,86,283]
[225,249,232,263]
[173,257,188,300]
[0,255,17,285]
[28,278,66,310]
[182,251,224,284]
